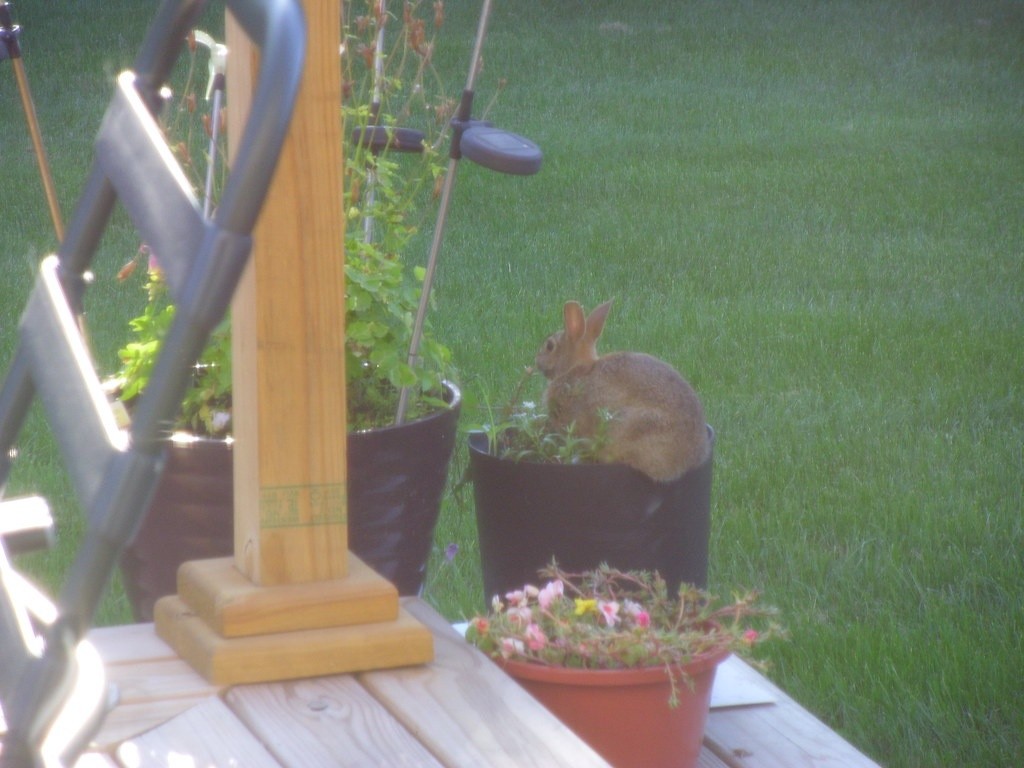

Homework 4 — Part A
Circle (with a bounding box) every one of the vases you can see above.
[493,613,733,768]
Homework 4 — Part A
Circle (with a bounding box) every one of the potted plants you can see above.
[467,374,714,616]
[84,0,460,621]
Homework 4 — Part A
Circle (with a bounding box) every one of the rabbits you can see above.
[535,296,711,484]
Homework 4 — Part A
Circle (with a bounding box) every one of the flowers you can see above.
[466,554,790,709]
